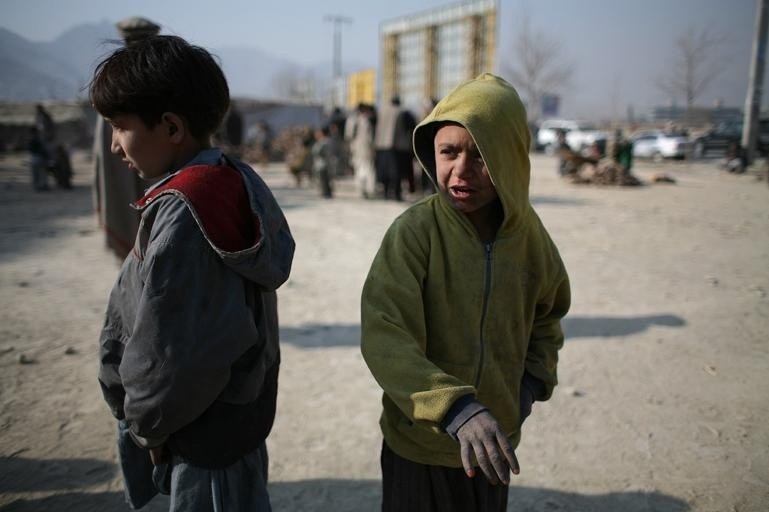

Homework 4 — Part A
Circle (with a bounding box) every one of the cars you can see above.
[530,115,769,165]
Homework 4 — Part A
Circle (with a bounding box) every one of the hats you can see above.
[116,18,161,38]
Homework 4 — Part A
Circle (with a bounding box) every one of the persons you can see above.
[92,16,160,257]
[211,101,246,161]
[556,127,632,186]
[295,93,438,203]
[90,35,295,512]
[255,118,272,164]
[29,104,75,191]
[361,73,570,512]
